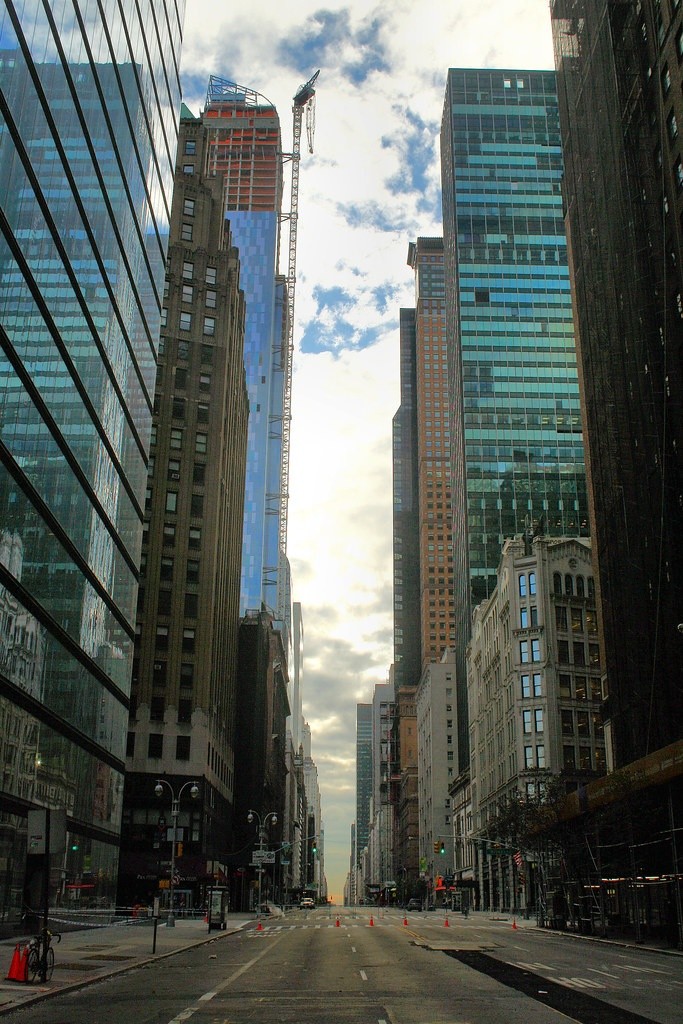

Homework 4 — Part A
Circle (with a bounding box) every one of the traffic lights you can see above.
[434,842,439,853]
[519,872,524,885]
[72,840,78,850]
[313,842,316,851]
[252,881,259,887]
[176,843,182,857]
[440,842,444,853]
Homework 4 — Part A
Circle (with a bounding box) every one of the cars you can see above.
[424,902,435,912]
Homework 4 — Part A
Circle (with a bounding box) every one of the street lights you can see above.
[247,809,278,930]
[155,779,200,928]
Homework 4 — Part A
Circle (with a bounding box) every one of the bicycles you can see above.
[25,928,61,983]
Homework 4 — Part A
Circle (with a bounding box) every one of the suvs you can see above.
[299,898,315,910]
[406,899,422,910]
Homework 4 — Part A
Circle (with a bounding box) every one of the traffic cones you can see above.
[335,917,342,927]
[369,915,374,926]
[403,912,409,926]
[5,943,29,983]
[511,920,517,929]
[255,920,264,931]
[444,918,449,927]
